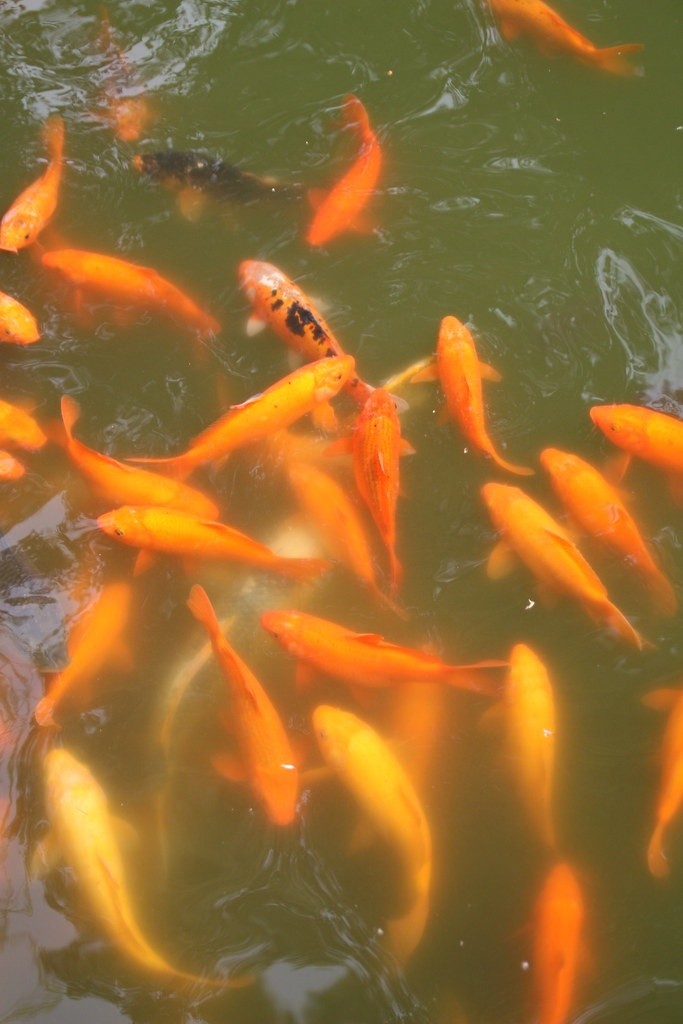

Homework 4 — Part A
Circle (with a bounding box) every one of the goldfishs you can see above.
[0,0,683,1023]
